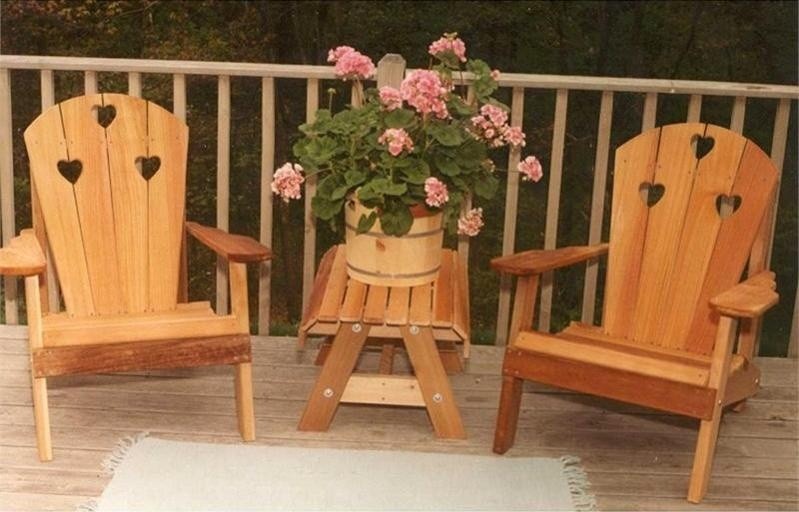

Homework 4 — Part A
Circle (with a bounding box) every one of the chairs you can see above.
[489,123,781,506]
[0,91,276,463]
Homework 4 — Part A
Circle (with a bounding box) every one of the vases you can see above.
[342,195,445,290]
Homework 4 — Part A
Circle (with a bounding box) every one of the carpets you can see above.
[75,431,593,511]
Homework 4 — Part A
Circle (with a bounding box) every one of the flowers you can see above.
[268,27,546,239]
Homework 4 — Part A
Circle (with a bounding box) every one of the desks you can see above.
[299,242,471,441]
